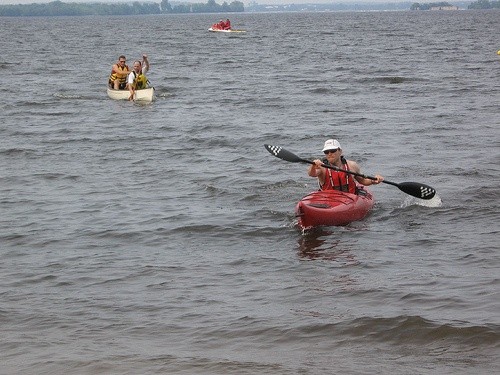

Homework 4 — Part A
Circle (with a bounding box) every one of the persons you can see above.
[109,55,132,89]
[127,54,149,101]
[212,18,231,30]
[307,139,384,196]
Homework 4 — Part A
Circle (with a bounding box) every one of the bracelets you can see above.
[371,179,373,184]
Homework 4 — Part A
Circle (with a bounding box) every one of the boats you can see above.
[107,87,156,102]
[208,27,246,33]
[294,184,375,228]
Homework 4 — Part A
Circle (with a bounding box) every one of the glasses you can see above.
[323,149,338,154]
[120,61,125,63]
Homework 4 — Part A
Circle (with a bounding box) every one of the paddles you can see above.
[128,58,145,102]
[263,143,437,201]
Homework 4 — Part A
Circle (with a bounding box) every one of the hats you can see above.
[321,139,341,151]
